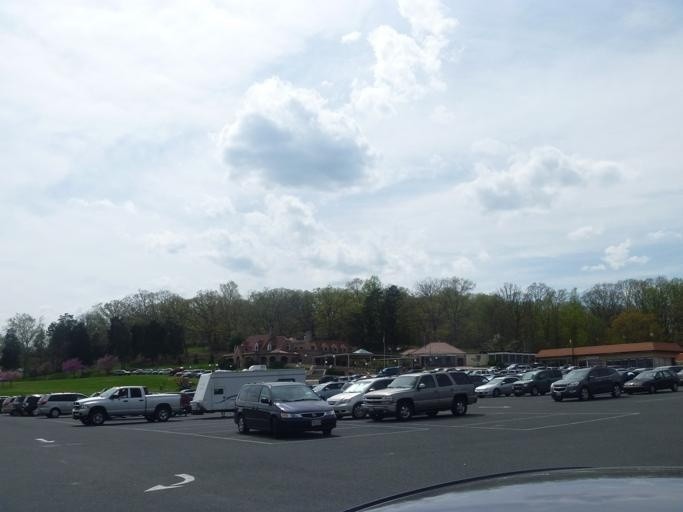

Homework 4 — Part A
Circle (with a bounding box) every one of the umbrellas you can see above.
[353,349,373,355]
